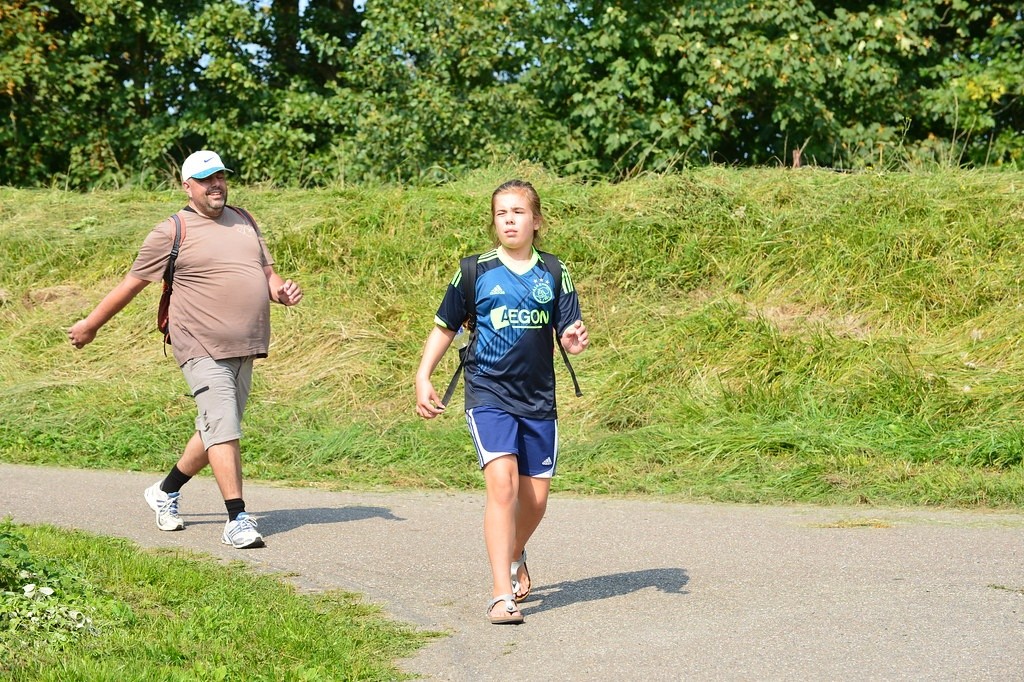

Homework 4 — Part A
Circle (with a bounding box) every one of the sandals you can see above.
[485,593,524,625]
[513,546,532,603]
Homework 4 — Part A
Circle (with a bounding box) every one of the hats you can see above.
[182,150,234,183]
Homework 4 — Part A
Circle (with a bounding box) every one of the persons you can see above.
[416,179,590,624]
[67,150,303,549]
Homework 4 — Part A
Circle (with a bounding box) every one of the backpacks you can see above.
[157,204,258,358]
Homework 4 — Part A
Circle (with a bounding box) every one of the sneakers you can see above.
[221,511,265,549]
[142,479,185,531]
